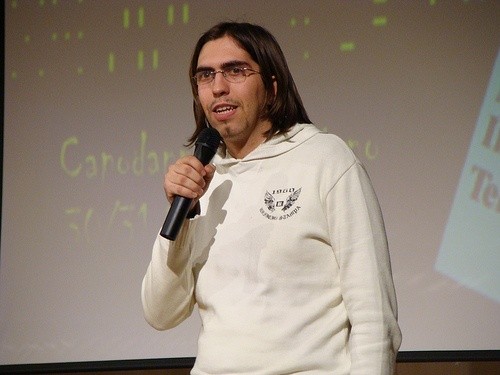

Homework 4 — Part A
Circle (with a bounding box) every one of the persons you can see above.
[142,22,402,375]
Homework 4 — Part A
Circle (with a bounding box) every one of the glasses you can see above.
[192,66,261,85]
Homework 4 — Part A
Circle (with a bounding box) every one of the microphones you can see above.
[160,127,221,241]
